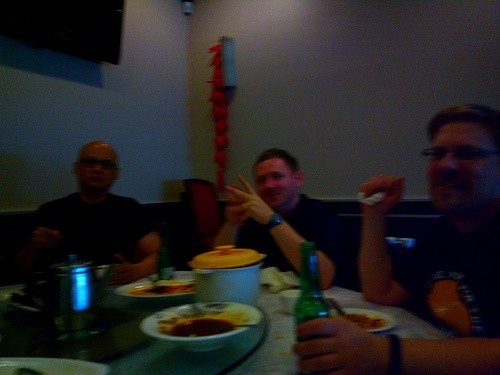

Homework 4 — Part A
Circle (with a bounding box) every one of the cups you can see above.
[278,288,301,315]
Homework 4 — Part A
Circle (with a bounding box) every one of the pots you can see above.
[188,245,266,306]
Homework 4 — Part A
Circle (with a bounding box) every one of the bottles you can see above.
[293,240,338,375]
[42,252,98,315]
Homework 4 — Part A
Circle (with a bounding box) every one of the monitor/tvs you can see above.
[1,0,126,65]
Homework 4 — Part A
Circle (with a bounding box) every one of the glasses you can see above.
[420,147,500,161]
[78,157,116,172]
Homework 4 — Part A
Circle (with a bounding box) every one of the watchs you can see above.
[264,214,283,231]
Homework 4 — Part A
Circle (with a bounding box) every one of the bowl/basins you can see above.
[93,264,117,281]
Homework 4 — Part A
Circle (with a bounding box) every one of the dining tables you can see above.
[0,264,454,375]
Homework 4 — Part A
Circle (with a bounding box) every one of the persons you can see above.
[14,140,163,285]
[210,148,342,290]
[290,103,500,375]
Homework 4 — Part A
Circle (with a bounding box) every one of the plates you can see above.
[340,308,398,333]
[140,300,264,354]
[113,271,196,297]
[0,283,42,314]
[0,357,110,375]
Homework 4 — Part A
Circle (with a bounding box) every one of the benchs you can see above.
[0,198,445,294]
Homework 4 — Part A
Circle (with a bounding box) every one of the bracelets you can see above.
[388,334,402,375]
